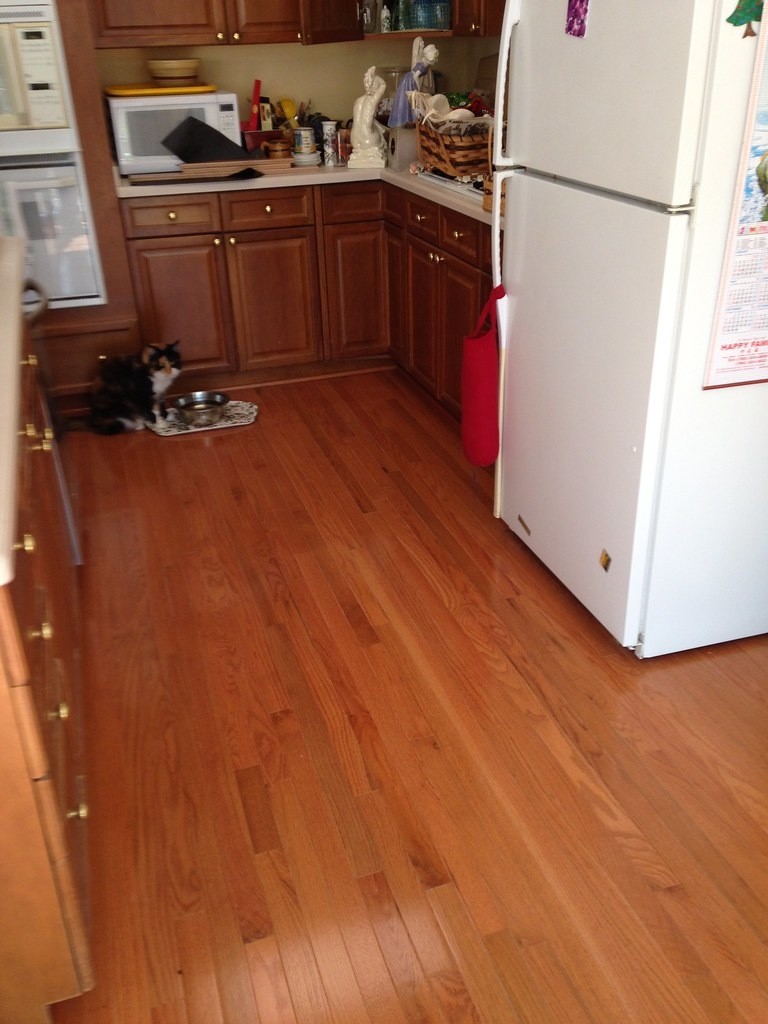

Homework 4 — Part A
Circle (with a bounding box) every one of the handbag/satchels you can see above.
[459,283,506,469]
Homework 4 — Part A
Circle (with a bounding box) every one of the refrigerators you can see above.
[486,0,768,659]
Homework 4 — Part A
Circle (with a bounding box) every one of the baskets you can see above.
[482,125,506,215]
[415,112,508,185]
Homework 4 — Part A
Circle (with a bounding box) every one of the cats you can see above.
[89,337,183,436]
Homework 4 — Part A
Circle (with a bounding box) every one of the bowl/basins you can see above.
[146,58,203,87]
[170,391,230,427]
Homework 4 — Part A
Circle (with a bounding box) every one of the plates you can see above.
[292,150,322,167]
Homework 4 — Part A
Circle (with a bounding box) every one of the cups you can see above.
[260,139,289,159]
[394,0,453,30]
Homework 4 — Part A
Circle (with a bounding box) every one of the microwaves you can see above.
[105,91,243,176]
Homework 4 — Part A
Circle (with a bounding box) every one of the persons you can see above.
[350,66,388,149]
[387,45,440,127]
[381,5,390,32]
[413,37,436,94]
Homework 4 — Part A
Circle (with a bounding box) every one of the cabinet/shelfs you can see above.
[119,177,502,423]
[0,313,96,1024]
[88,0,501,49]
